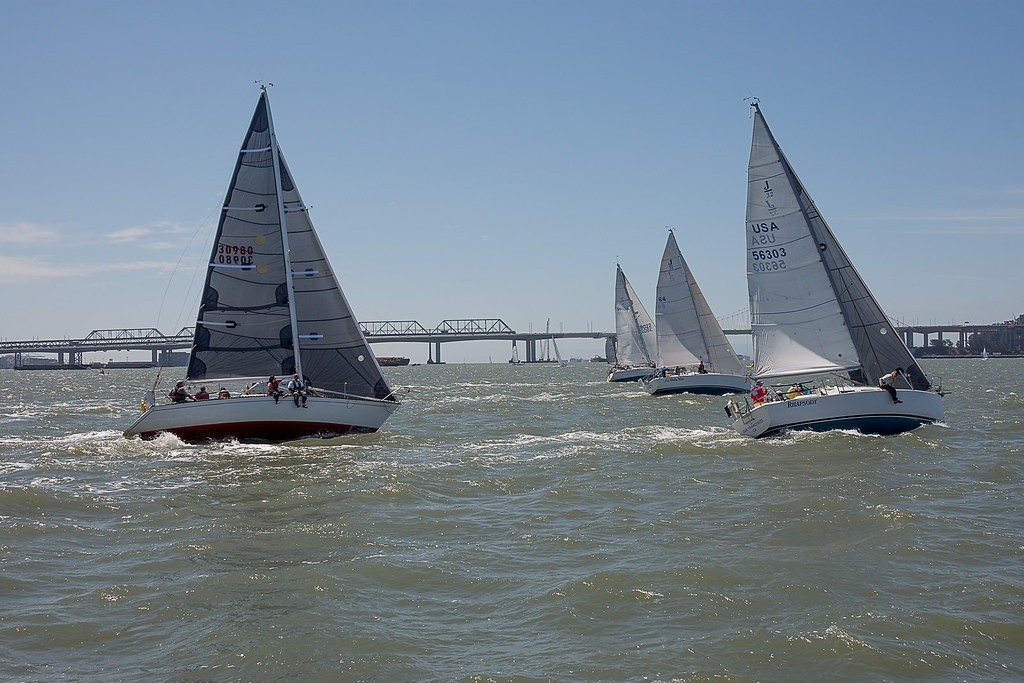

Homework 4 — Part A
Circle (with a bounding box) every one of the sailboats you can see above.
[729,96,945,440]
[608,263,658,384]
[123,80,400,444]
[642,228,751,397]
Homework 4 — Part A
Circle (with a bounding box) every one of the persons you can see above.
[785,382,813,399]
[167,379,199,403]
[750,380,768,408]
[267,374,281,405]
[616,361,708,378]
[287,374,309,409]
[218,386,231,400]
[195,385,209,401]
[878,370,903,405]
